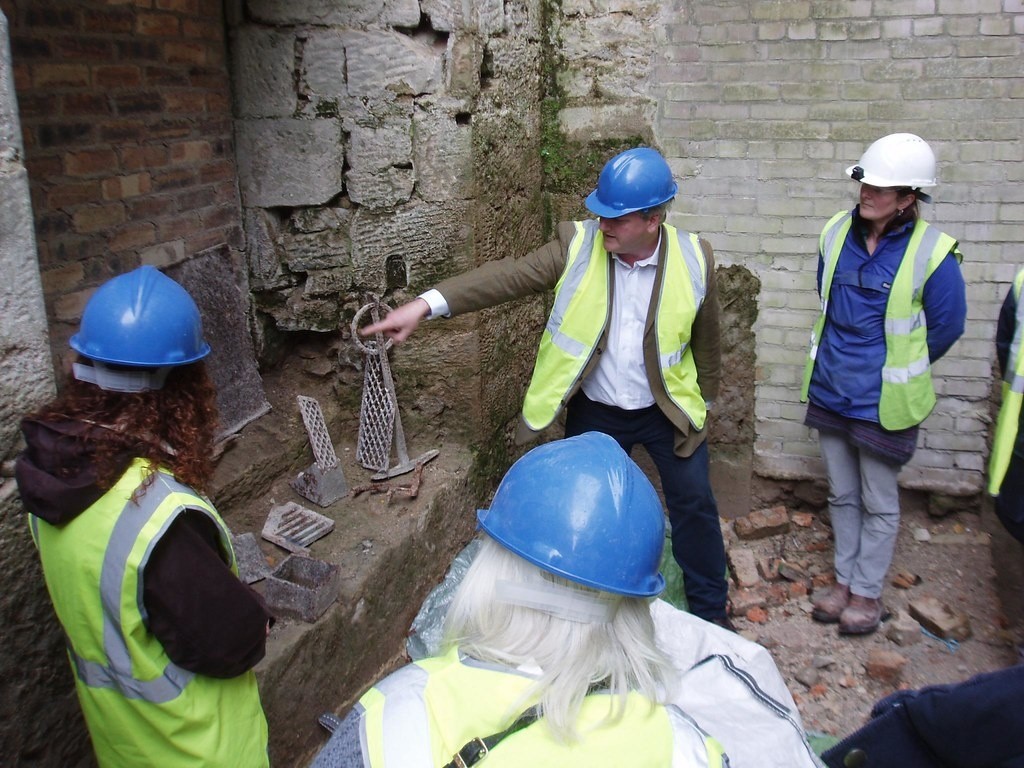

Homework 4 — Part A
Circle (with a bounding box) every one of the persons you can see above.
[360,147,736,631]
[986,266,1023,541]
[800,132,966,631]
[310,430,731,768]
[16,265,276,768]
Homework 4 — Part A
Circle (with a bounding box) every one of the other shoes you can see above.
[707,617,737,634]
[812,582,850,621]
[839,595,884,633]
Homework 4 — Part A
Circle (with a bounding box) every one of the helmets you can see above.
[70,266,210,365]
[475,430,665,597]
[845,133,937,188]
[585,147,678,219]
